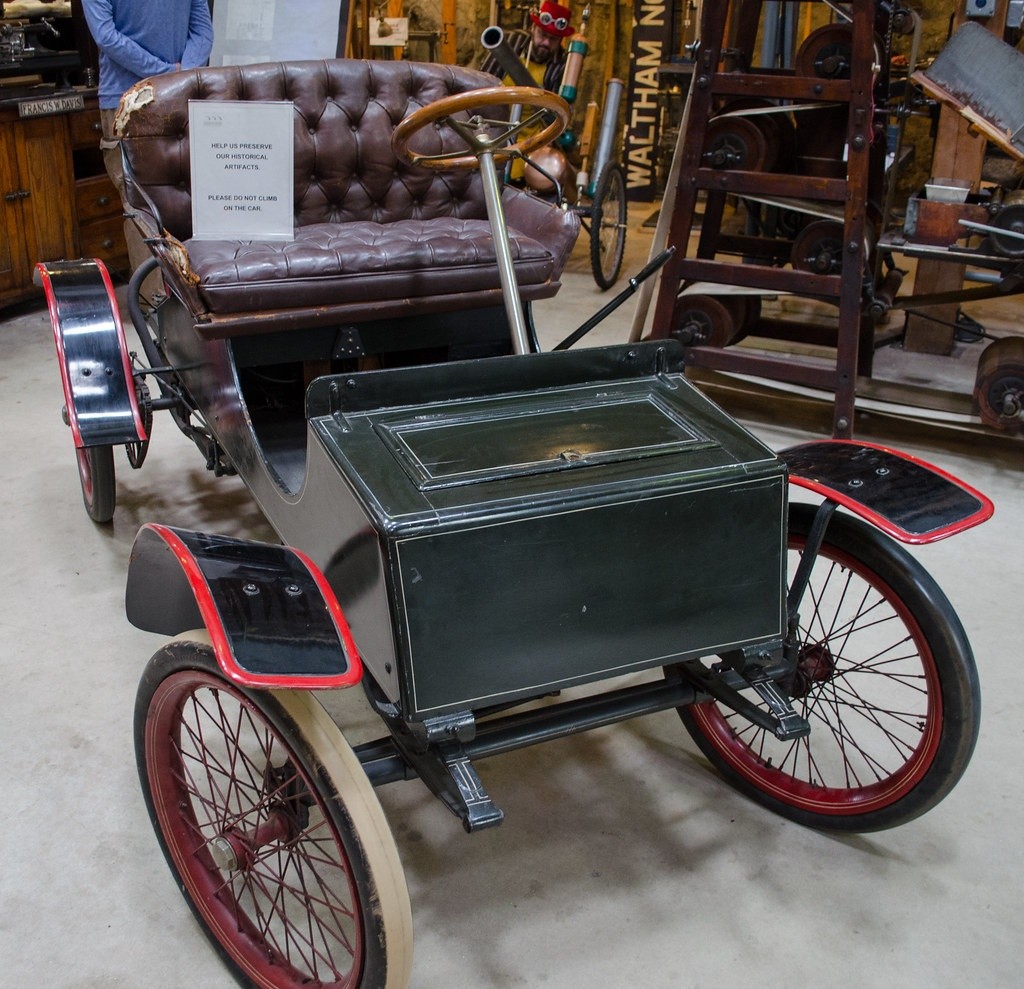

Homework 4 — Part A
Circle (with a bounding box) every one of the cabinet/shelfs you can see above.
[0,83,132,310]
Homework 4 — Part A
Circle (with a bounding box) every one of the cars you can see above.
[31,57,997,989]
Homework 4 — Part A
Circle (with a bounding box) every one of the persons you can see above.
[81,0,214,318]
[502,0,575,190]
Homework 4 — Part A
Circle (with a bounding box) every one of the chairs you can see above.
[115,59,583,337]
